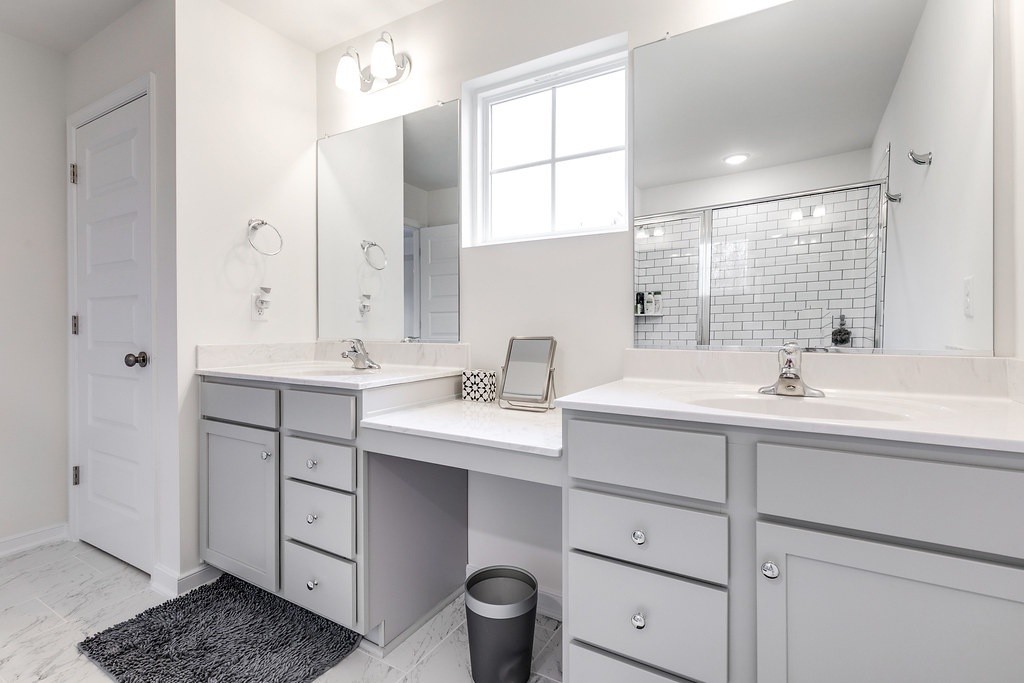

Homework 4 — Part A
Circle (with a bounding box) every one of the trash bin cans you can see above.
[464,564,538,683]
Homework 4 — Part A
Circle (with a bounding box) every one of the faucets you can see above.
[778,341,803,378]
[341,351,360,367]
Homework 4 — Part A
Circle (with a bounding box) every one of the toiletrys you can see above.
[645,293,654,314]
[636,292,645,316]
[654,291,662,314]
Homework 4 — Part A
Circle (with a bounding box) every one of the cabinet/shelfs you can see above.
[560,406,1024,683]
[199,372,467,660]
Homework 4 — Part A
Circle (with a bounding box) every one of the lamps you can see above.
[334,31,412,96]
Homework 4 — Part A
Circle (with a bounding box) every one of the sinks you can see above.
[680,393,911,433]
[281,368,407,385]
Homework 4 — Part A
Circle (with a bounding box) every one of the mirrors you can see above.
[315,97,461,345]
[628,0,995,356]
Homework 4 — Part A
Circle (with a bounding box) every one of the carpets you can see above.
[75,574,366,683]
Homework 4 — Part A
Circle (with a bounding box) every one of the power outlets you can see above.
[355,299,367,322]
[251,293,269,321]
